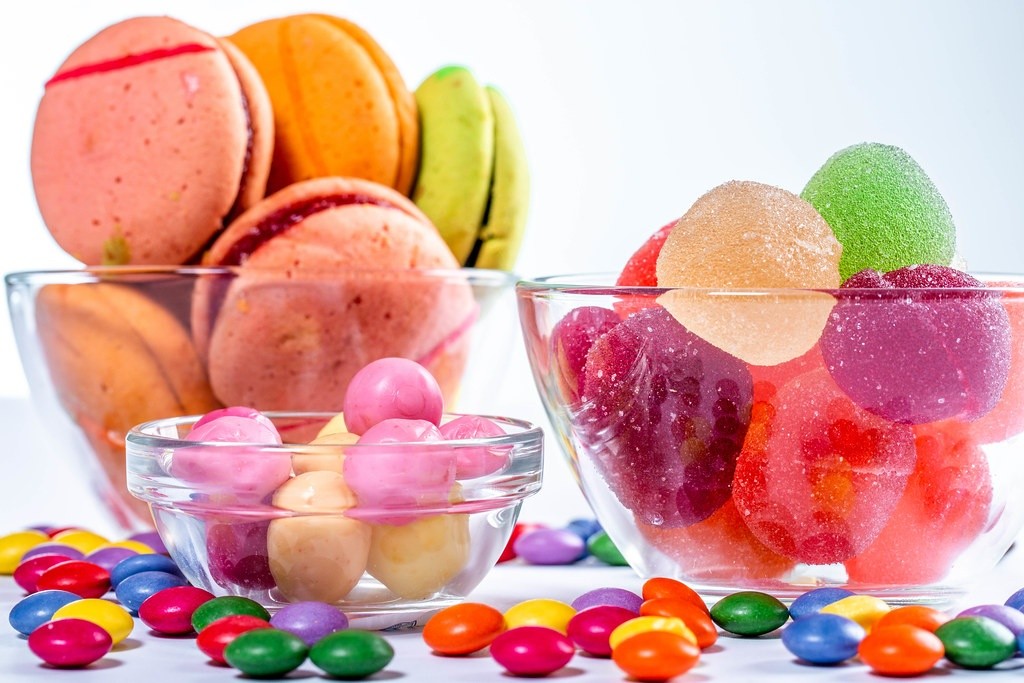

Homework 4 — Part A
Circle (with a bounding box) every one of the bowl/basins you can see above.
[515,274,1023,619]
[4,268,585,537]
[123,412,545,631]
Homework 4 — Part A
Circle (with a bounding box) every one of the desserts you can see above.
[35,12,526,531]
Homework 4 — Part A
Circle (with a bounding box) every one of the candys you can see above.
[0,140,1024,683]
